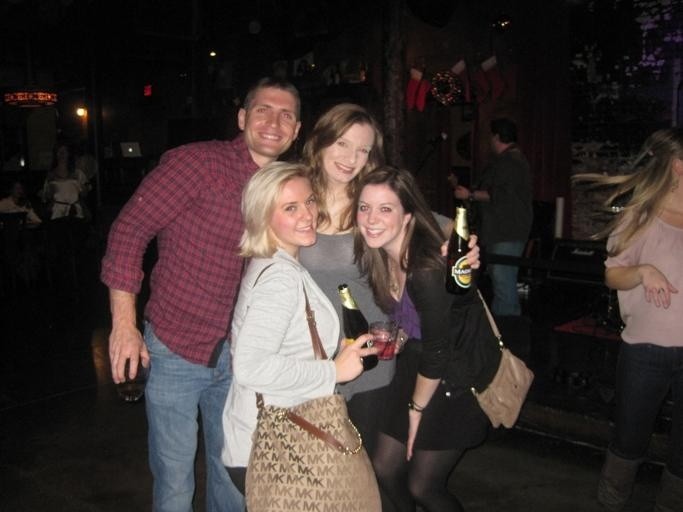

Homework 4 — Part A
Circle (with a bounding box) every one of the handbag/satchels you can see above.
[246,395,382,512]
[478,350,535,431]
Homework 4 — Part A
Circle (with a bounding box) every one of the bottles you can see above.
[446,204,473,295]
[336,283,379,371]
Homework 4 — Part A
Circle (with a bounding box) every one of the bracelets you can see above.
[407,400,425,412]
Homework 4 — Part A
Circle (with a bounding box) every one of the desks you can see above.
[546,236,626,335]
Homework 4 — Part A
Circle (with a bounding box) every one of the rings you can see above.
[658,288,664,294]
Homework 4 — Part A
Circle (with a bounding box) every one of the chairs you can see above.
[521,236,624,384]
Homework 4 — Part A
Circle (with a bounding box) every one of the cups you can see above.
[116,359,152,402]
[368,319,401,360]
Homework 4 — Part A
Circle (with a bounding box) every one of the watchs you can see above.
[469,190,474,203]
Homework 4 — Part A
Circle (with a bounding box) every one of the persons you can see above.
[289,103,484,436]
[221,161,382,512]
[354,166,508,512]
[597,126,682,512]
[101,76,301,511]
[41,143,93,237]
[1,182,41,225]
[455,117,535,316]
